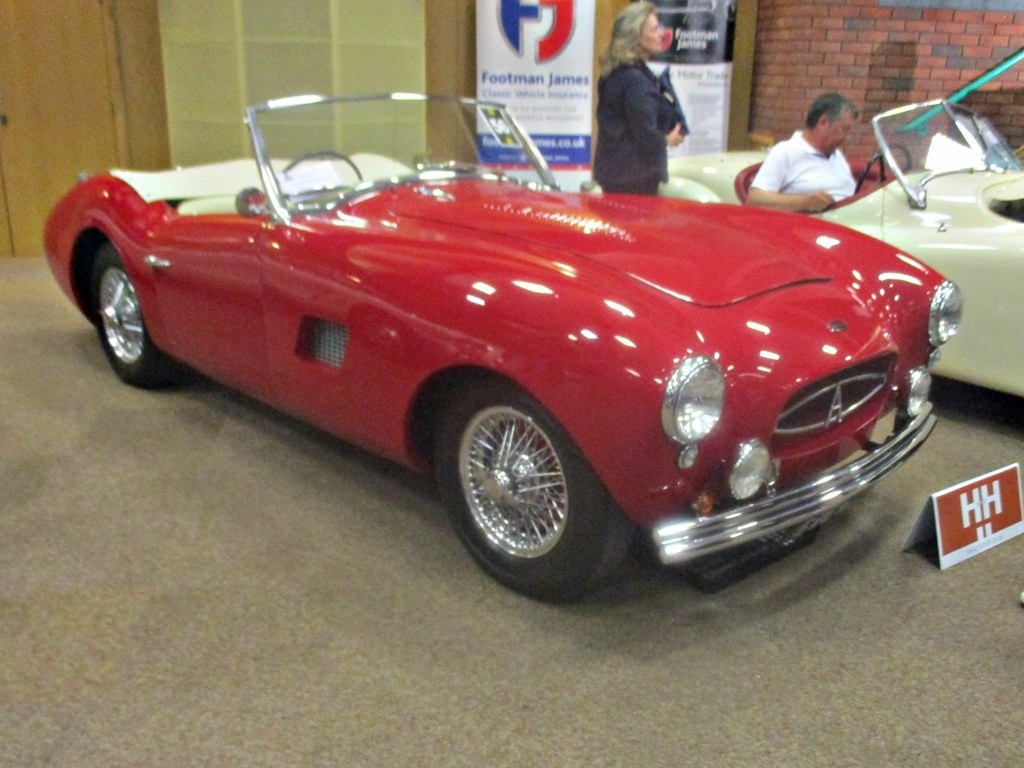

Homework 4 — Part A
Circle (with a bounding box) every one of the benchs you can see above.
[735,156,902,215]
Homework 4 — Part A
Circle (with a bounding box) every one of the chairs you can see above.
[177,195,238,220]
[341,151,410,187]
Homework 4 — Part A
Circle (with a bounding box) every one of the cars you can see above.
[42,93,963,606]
[579,99,1024,404]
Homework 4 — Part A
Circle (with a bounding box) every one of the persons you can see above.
[747,93,858,207]
[593,2,684,196]
